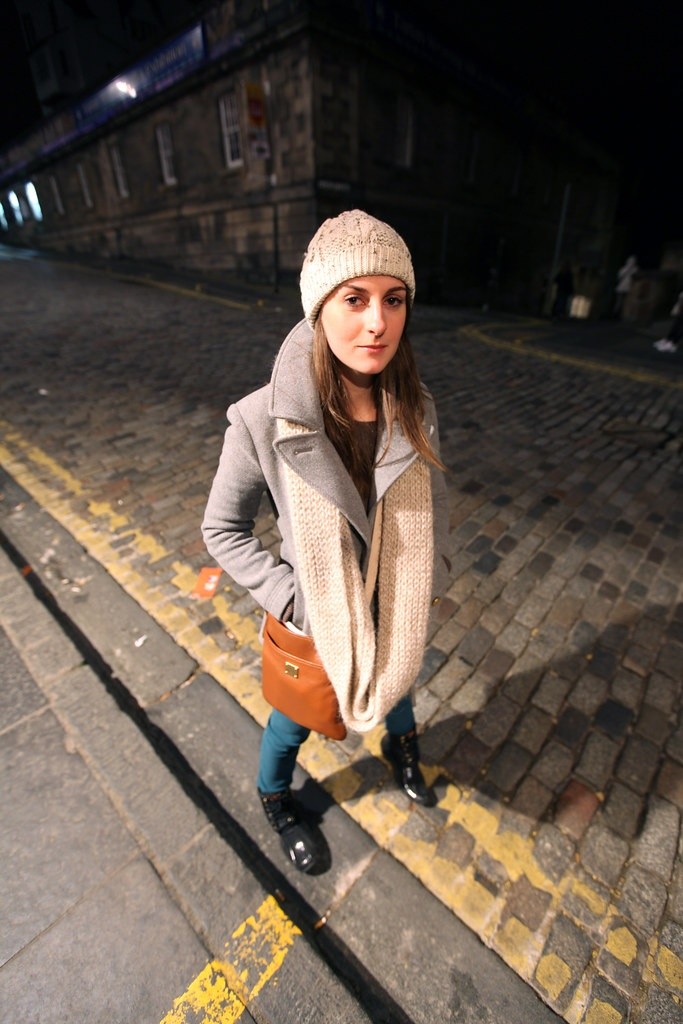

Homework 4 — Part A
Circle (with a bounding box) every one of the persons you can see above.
[200,208,454,876]
[654,295,683,352]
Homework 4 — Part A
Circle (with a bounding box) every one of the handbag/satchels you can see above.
[261,614,349,740]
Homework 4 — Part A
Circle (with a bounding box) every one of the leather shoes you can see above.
[257,786,327,872]
[382,725,433,803]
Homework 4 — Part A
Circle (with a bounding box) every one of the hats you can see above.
[299,209,416,332]
